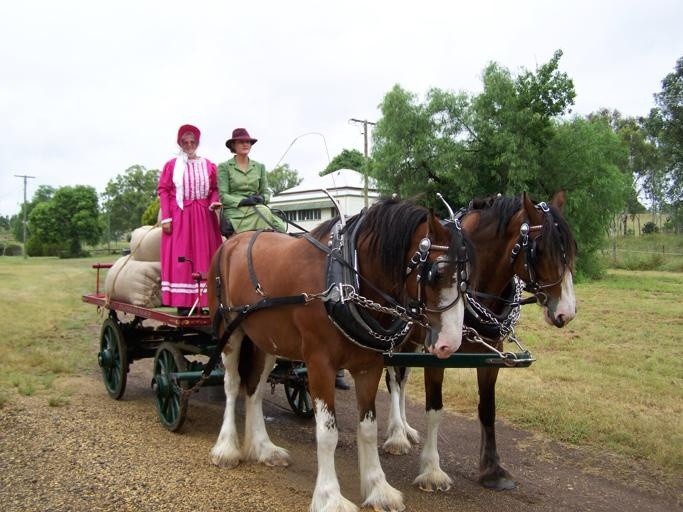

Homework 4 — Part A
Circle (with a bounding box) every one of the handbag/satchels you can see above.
[220,218,234,236]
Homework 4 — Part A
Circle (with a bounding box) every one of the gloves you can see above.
[239,195,265,206]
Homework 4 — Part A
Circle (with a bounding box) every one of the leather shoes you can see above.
[178,307,190,315]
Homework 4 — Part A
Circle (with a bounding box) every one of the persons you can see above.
[214,126,287,236]
[333,368,350,391]
[156,125,224,315]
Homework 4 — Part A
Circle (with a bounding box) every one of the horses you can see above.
[208,190,482,512]
[381,185,578,491]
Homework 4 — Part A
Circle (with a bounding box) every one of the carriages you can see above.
[79,180,578,512]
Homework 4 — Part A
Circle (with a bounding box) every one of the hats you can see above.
[177,125,200,148]
[226,128,257,153]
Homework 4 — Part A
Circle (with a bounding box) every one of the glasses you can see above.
[182,141,196,147]
